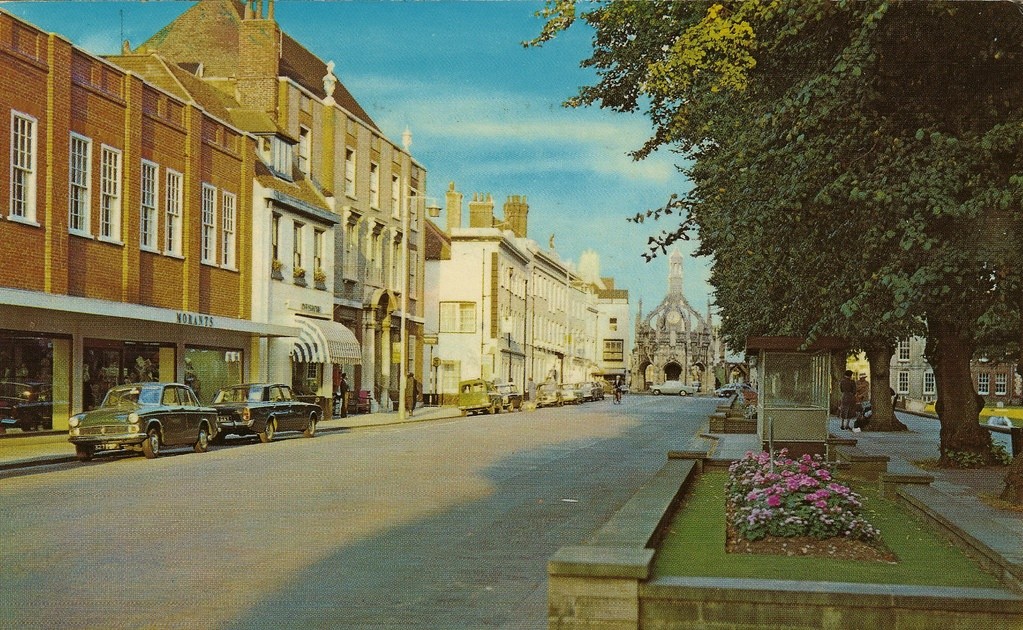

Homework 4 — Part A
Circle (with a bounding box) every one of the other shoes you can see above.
[840,425,845,430]
[844,426,852,430]
[409,410,413,416]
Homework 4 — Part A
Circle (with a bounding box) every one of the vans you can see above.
[457,379,504,418]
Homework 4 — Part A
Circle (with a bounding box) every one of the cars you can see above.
[559,384,584,405]
[649,380,694,396]
[0,379,54,431]
[210,381,323,444]
[536,382,564,408]
[580,381,604,402]
[67,381,219,462]
[714,384,758,398]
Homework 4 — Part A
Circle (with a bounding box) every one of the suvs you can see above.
[494,383,524,412]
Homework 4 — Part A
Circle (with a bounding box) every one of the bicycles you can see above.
[613,386,621,404]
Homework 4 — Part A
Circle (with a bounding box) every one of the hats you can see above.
[859,373,868,379]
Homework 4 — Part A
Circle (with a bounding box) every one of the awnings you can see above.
[291,316,362,366]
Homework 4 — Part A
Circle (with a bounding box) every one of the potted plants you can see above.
[272,258,285,280]
[314,268,327,290]
[293,266,307,287]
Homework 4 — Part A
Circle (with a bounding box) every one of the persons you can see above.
[405,372,417,418]
[839,370,870,430]
[613,375,623,402]
[340,373,350,417]
[527,377,536,403]
